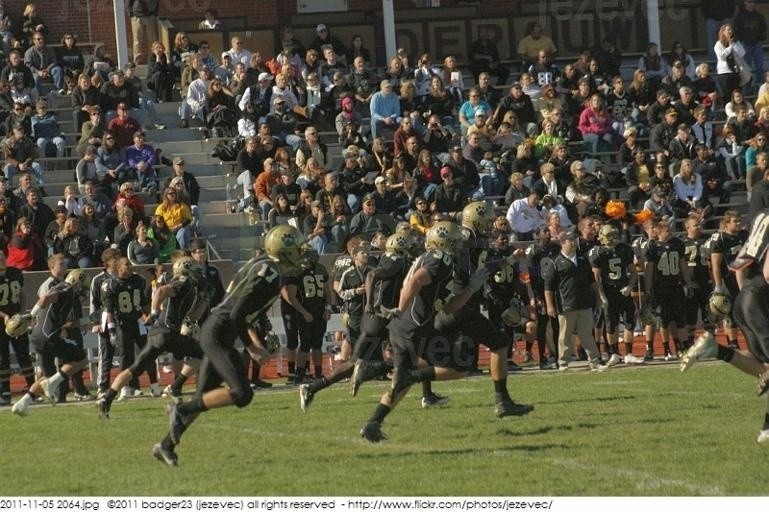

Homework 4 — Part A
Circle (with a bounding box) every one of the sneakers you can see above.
[286,374,323,411]
[181,119,188,128]
[664,351,684,361]
[756,370,768,395]
[507,349,652,371]
[495,401,534,417]
[250,381,273,389]
[757,429,769,443]
[349,359,451,442]
[1,378,184,419]
[153,403,187,470]
[153,123,167,129]
[680,332,719,373]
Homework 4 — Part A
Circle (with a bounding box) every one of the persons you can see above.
[0,0,769,466]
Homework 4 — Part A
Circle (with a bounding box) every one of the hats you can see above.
[86,145,101,157]
[559,231,580,242]
[222,52,231,59]
[363,176,388,204]
[173,156,186,165]
[475,109,487,117]
[441,167,451,177]
[665,107,679,115]
[273,97,287,106]
[117,102,128,109]
[199,65,209,71]
[381,80,392,88]
[316,24,326,32]
[448,143,461,153]
[258,73,274,81]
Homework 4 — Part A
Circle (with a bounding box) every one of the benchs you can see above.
[1,50,769,276]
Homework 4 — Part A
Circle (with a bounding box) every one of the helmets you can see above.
[638,300,658,326]
[709,291,732,316]
[172,257,204,286]
[599,224,620,248]
[386,201,496,257]
[266,225,319,270]
[66,270,92,302]
[265,330,281,354]
[501,297,529,327]
[5,314,33,338]
[181,323,200,342]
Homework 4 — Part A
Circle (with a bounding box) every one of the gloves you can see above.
[108,323,119,348]
[600,295,609,309]
[620,286,633,297]
[467,268,490,294]
[376,305,402,321]
[142,312,159,326]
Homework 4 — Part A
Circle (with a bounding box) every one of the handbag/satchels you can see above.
[734,53,752,88]
